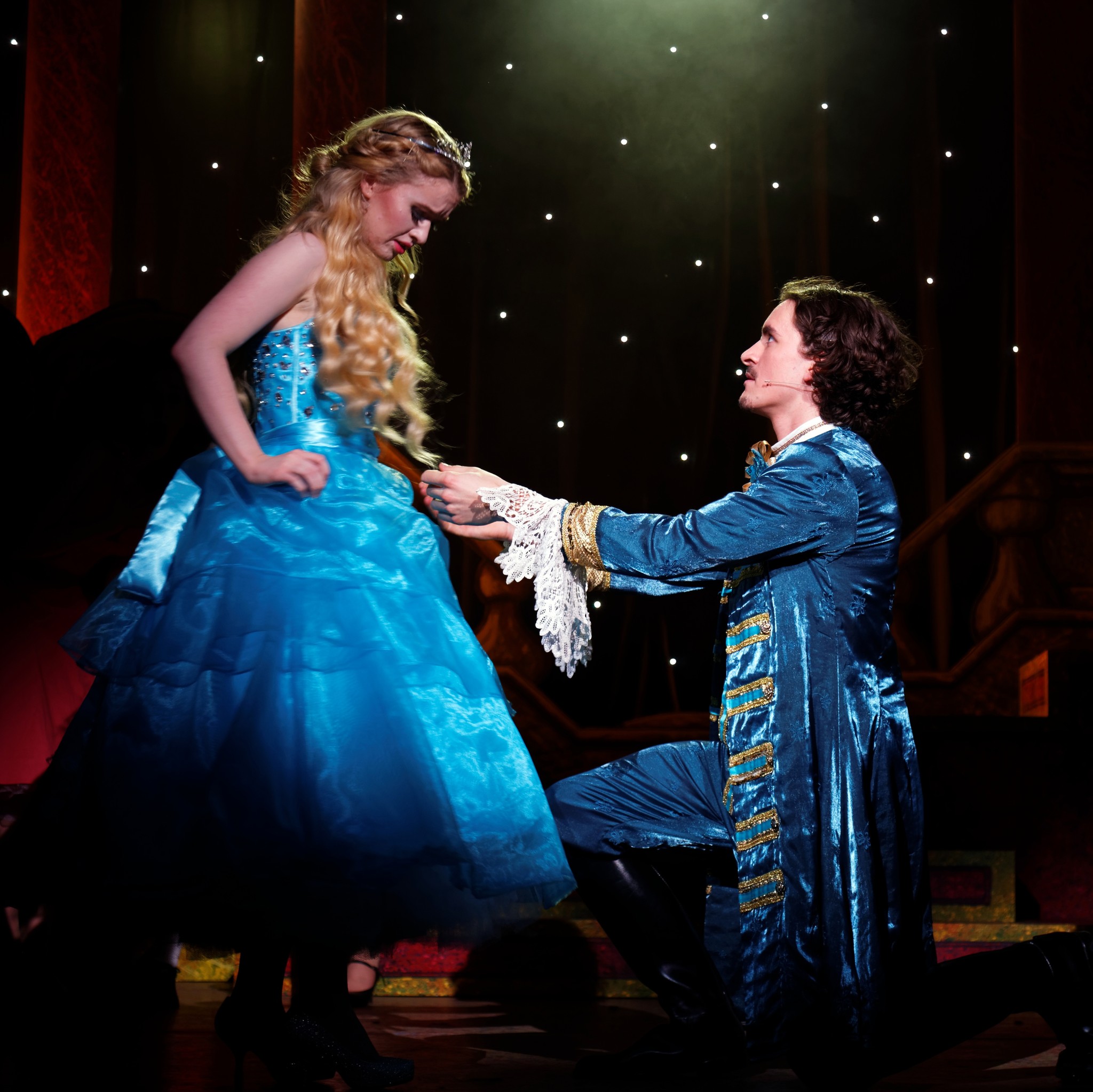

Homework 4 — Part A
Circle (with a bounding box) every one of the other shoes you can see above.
[348,959,379,1004]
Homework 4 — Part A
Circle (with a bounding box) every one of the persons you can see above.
[414,275,1093,1084]
[0,108,575,1092]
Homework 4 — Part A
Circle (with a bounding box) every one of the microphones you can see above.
[765,380,818,389]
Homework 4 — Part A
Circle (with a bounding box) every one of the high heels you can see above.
[215,1001,334,1081]
[290,992,415,1092]
